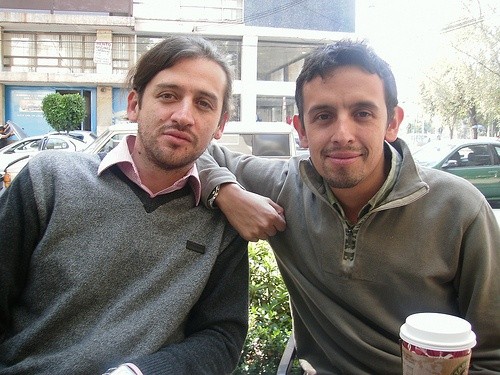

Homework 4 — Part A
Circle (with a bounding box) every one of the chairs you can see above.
[448,152,490,166]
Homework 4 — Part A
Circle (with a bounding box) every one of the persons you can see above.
[193,36,500,375]
[0,123,15,149]
[0,36,249,375]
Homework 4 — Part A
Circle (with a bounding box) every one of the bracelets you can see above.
[102,366,137,375]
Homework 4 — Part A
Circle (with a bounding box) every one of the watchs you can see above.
[207,185,220,210]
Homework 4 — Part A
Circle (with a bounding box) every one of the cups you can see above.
[399,312,477,375]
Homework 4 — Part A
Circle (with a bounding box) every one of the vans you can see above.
[82,121,296,164]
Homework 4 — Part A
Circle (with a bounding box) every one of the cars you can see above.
[1,135,89,173]
[412,139,500,200]
[6,120,98,147]
